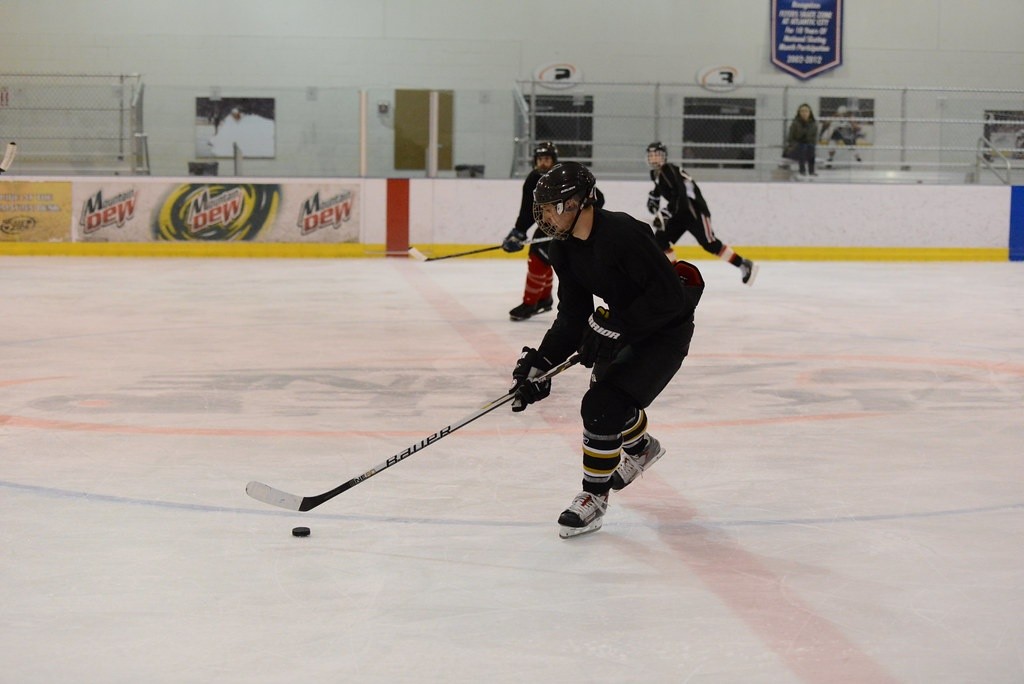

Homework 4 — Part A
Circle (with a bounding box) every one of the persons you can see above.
[508,160,706,537]
[782,104,819,176]
[503,141,562,320]
[643,140,760,288]
[820,106,872,169]
[208,102,222,133]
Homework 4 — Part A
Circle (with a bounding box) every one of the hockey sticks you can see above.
[653,208,667,232]
[244,349,580,514]
[408,234,554,263]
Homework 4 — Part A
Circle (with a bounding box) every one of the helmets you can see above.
[533,161,596,208]
[534,142,556,165]
[646,142,668,165]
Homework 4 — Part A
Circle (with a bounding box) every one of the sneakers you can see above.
[509,303,537,320]
[738,259,753,283]
[558,475,614,538]
[533,296,553,314]
[610,431,667,491]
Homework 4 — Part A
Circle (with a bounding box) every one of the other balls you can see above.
[291,527,310,537]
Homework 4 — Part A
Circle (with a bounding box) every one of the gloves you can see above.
[647,191,659,214]
[577,305,624,368]
[503,228,527,252]
[653,208,671,230]
[509,346,551,412]
